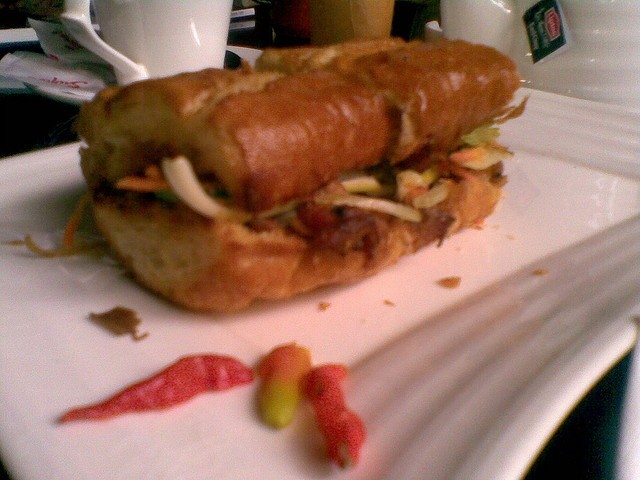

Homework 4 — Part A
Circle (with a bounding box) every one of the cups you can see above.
[59,1,233,88]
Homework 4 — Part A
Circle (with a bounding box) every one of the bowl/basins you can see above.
[26,13,102,63]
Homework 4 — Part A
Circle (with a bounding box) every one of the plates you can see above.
[23,45,264,106]
[0,87,639,479]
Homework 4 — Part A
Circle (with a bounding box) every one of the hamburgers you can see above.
[76,38,527,314]
